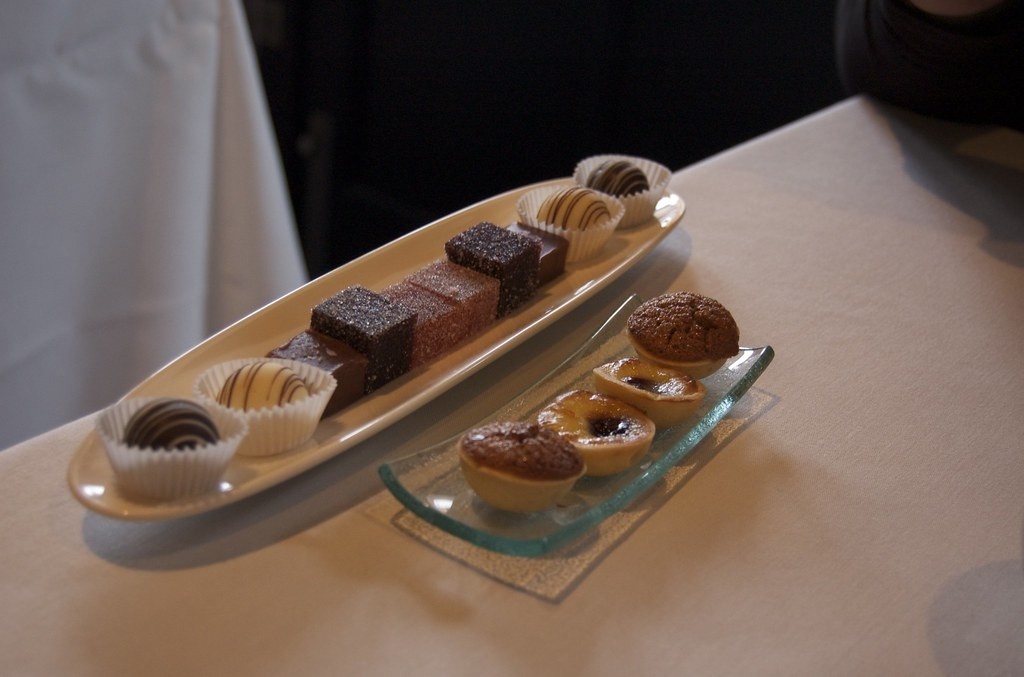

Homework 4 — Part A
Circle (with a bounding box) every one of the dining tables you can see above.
[0,93,1024,676]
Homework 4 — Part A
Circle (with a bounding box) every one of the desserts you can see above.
[455,289,741,512]
[94,155,671,497]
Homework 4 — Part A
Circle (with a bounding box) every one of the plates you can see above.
[66,175,687,519]
[376,292,775,561]
